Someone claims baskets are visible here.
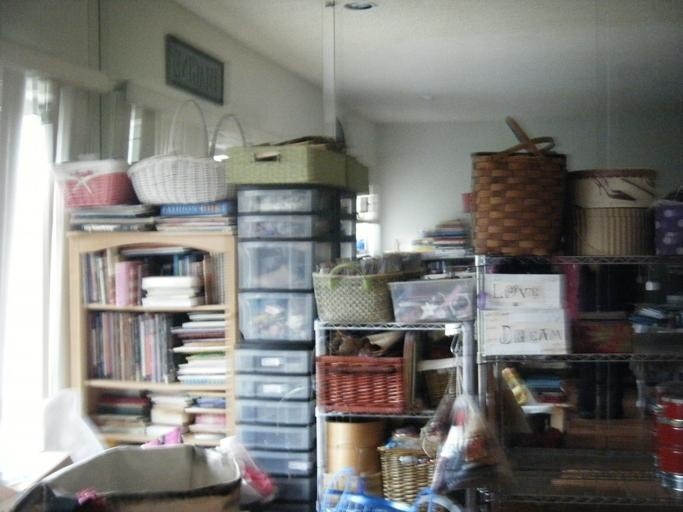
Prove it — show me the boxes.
[313,264,426,324]
[472,116,566,255]
[566,168,657,257]
[61,98,236,206]
[378,444,443,511]
[317,355,405,412]
[427,370,477,408]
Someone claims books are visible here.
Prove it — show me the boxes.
[67,202,238,446]
[413,218,474,255]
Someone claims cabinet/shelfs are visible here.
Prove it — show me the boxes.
[314,319,476,511]
[237,187,357,501]
[474,254,683,511]
[66,228,236,449]
